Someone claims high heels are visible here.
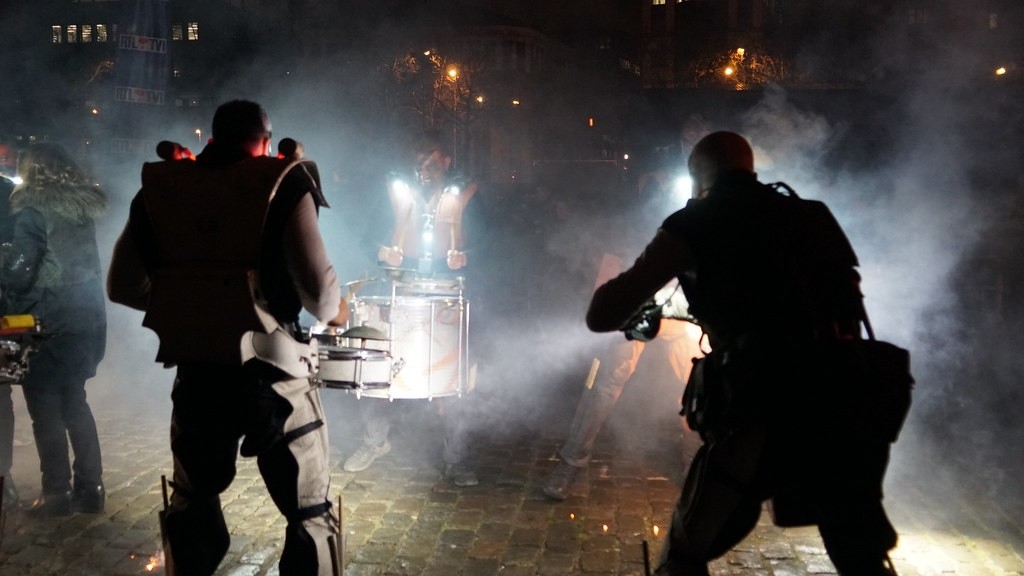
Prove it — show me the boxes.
[71,482,106,514]
[19,481,75,517]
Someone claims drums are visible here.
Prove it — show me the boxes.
[338,273,476,400]
[313,347,394,391]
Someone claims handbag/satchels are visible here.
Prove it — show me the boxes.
[0,329,86,398]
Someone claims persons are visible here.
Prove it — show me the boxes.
[539,105,781,502]
[584,129,901,575]
[106,97,350,576]
[341,131,483,487]
[0,141,108,523]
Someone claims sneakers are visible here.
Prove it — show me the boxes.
[342,436,391,471]
[543,458,580,500]
[445,461,478,487]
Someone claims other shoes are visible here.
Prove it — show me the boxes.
[1,474,19,509]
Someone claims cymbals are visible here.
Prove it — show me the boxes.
[338,325,393,342]
[308,325,351,345]
